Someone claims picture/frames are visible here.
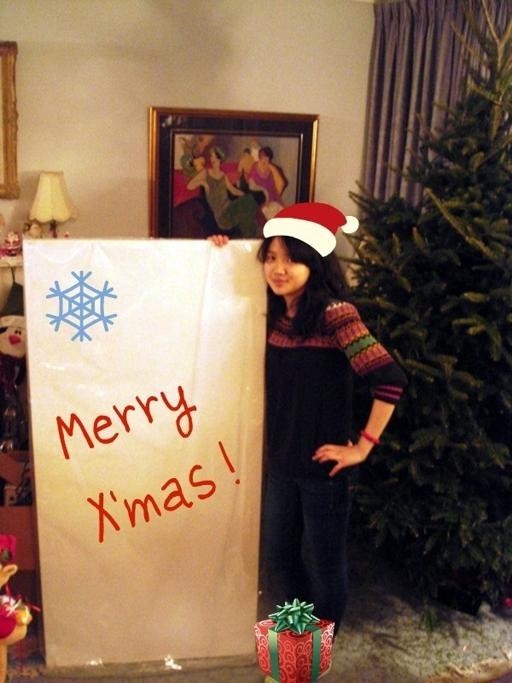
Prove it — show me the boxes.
[0,40,21,200]
[148,105,320,254]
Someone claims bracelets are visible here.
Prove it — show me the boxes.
[358,431,381,445]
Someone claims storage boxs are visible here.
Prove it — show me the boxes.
[0,451,38,576]
[253,614,335,683]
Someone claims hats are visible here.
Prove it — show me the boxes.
[262,202,360,258]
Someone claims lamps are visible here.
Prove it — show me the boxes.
[27,170,77,239]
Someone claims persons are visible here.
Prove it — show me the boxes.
[208,201,407,646]
[186,147,287,237]
